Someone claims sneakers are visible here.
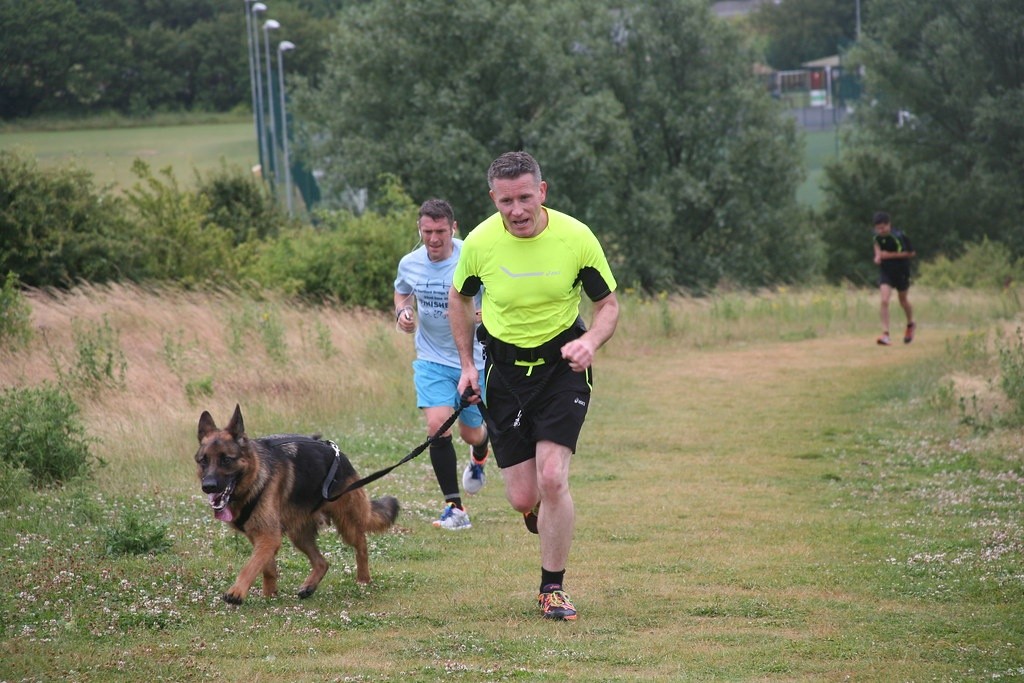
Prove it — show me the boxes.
[540,583,577,621]
[462,443,490,494]
[432,501,472,530]
[522,499,539,535]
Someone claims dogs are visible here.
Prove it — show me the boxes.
[195,404,400,605]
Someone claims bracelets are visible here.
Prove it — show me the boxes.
[397,309,404,321]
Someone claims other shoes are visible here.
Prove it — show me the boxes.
[877,334,890,345]
[904,323,917,343]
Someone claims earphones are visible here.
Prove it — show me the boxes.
[419,229,421,240]
[451,229,455,238]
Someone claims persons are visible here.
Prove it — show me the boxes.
[874,213,917,345]
[448,152,620,620]
[394,200,488,529]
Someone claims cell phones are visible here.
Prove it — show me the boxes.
[404,309,410,321]
